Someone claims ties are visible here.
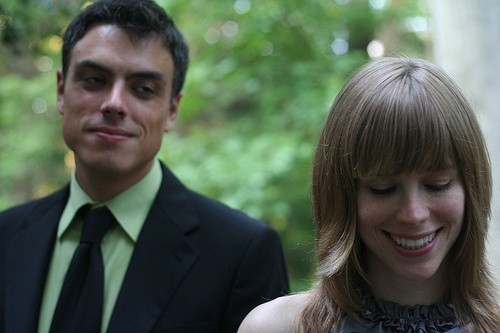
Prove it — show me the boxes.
[49,205,116,333]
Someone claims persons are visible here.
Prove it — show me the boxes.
[238,57,500,333]
[0,0,292,333]
